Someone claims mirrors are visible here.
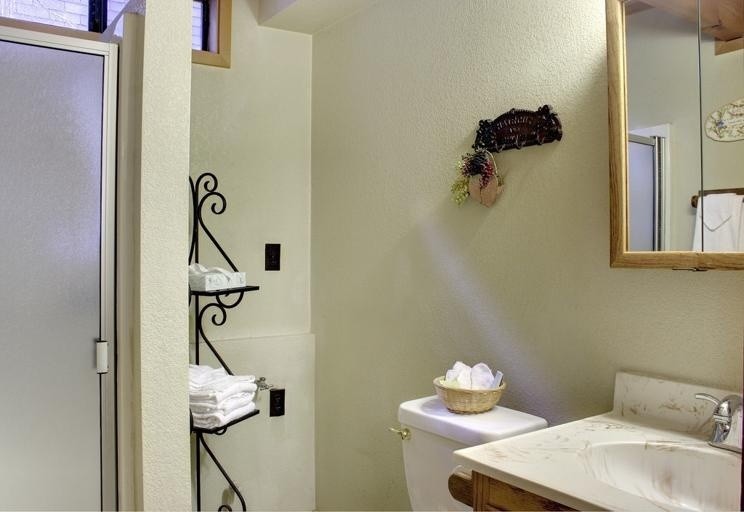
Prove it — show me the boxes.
[604,0,744,272]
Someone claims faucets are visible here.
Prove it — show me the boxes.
[710,395,743,454]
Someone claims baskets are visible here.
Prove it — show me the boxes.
[468,149,503,208]
[433,376,505,414]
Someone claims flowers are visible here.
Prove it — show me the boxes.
[450,146,495,209]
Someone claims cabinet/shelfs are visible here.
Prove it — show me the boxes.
[188,173,261,511]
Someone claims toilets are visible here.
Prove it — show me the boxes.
[389,396,549,512]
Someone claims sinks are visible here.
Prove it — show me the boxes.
[576,442,743,512]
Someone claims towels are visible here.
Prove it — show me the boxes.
[692,193,744,254]
[190,363,258,430]
